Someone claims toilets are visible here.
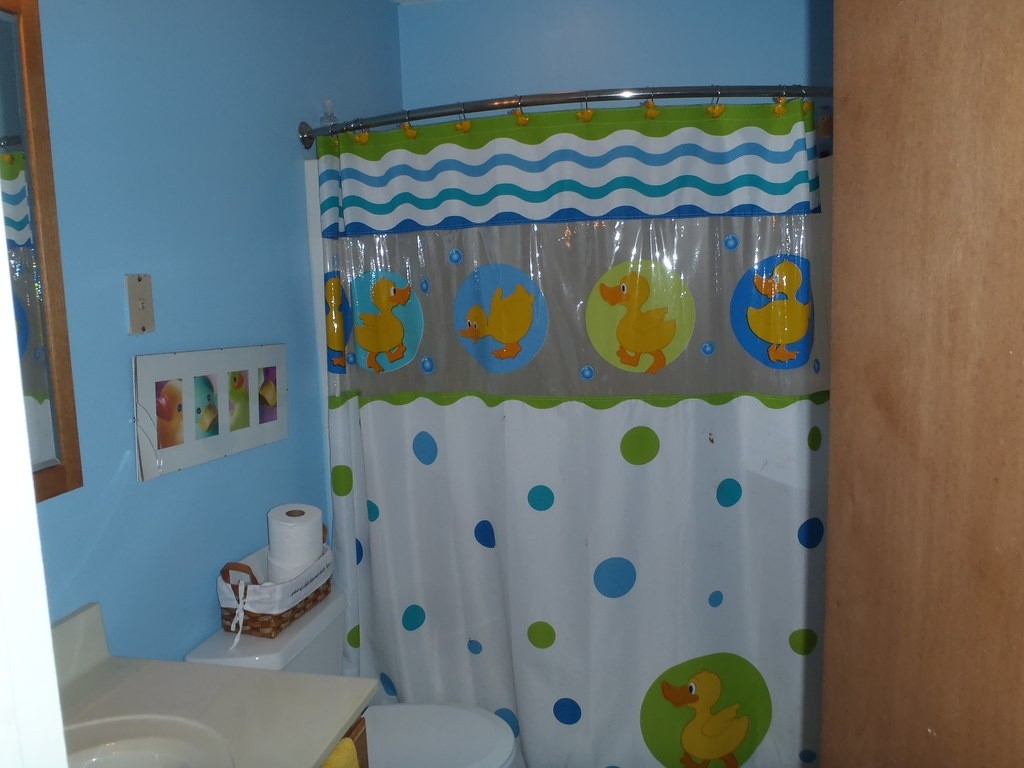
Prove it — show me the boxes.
[184,585,517,768]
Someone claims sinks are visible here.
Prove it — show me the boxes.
[62,711,238,768]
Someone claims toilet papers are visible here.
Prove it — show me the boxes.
[266,503,324,586]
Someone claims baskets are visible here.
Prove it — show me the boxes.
[217,523,333,637]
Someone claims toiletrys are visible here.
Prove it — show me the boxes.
[319,98,339,130]
